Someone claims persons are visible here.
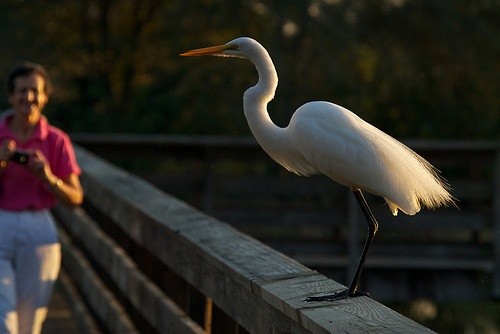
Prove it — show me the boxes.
[0,62,84,334]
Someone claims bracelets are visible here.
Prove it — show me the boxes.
[46,180,63,193]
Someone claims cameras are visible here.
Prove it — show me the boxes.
[9,149,29,164]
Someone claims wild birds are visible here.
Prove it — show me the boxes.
[179,37,462,303]
